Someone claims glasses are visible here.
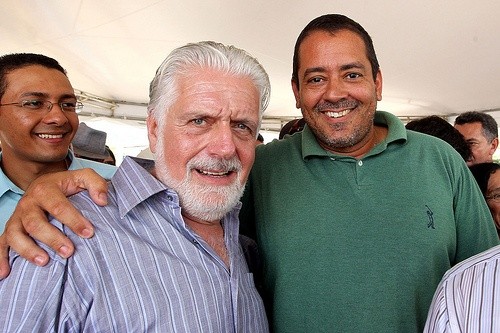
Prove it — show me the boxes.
[0,97,84,115]
[485,192,500,202]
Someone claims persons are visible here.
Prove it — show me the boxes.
[1,40,270,333]
[2,53,117,237]
[72,122,155,170]
[422,243,500,333]
[1,14,500,332]
[254,109,500,238]
